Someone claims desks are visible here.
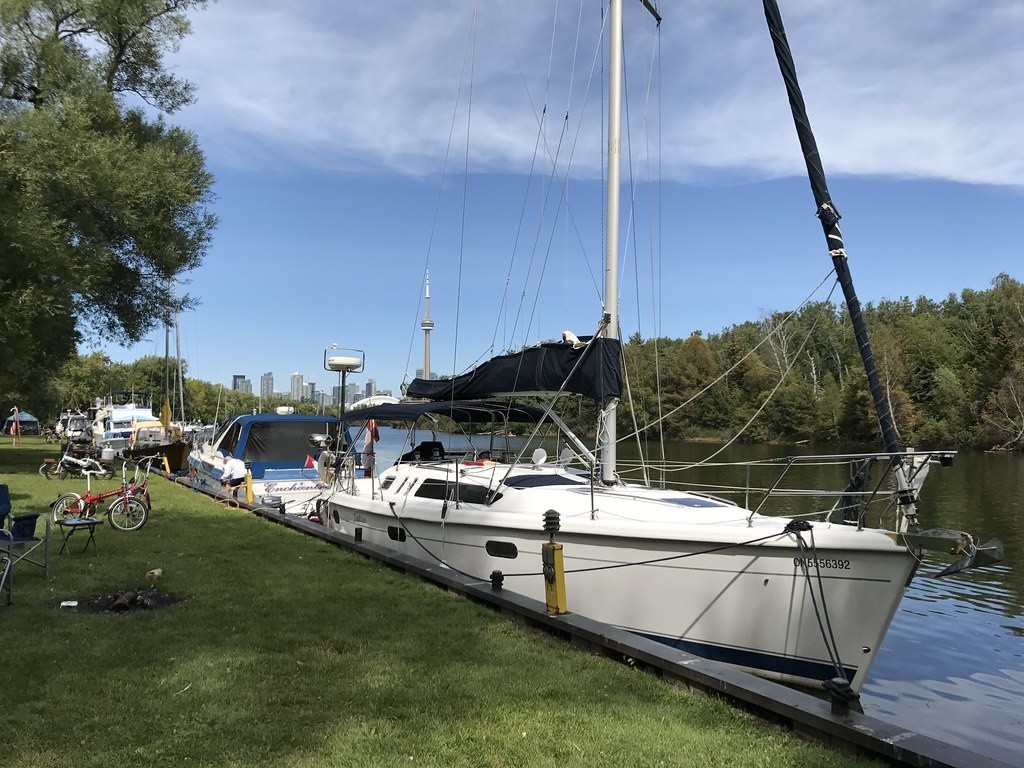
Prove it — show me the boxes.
[70,449,99,480]
[55,518,104,559]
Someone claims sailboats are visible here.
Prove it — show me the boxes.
[120,276,196,473]
[307,0,1006,705]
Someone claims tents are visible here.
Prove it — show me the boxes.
[3,411,40,436]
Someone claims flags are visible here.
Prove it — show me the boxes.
[367,419,380,442]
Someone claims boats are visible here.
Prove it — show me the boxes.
[39,379,222,481]
[186,383,381,521]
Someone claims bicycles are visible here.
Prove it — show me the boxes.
[116,452,165,517]
[39,439,115,482]
[50,451,149,531]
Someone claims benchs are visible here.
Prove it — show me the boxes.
[44,457,56,463]
[99,461,114,465]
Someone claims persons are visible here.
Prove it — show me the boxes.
[219,456,247,501]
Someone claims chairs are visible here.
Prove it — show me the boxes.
[0,484,51,607]
